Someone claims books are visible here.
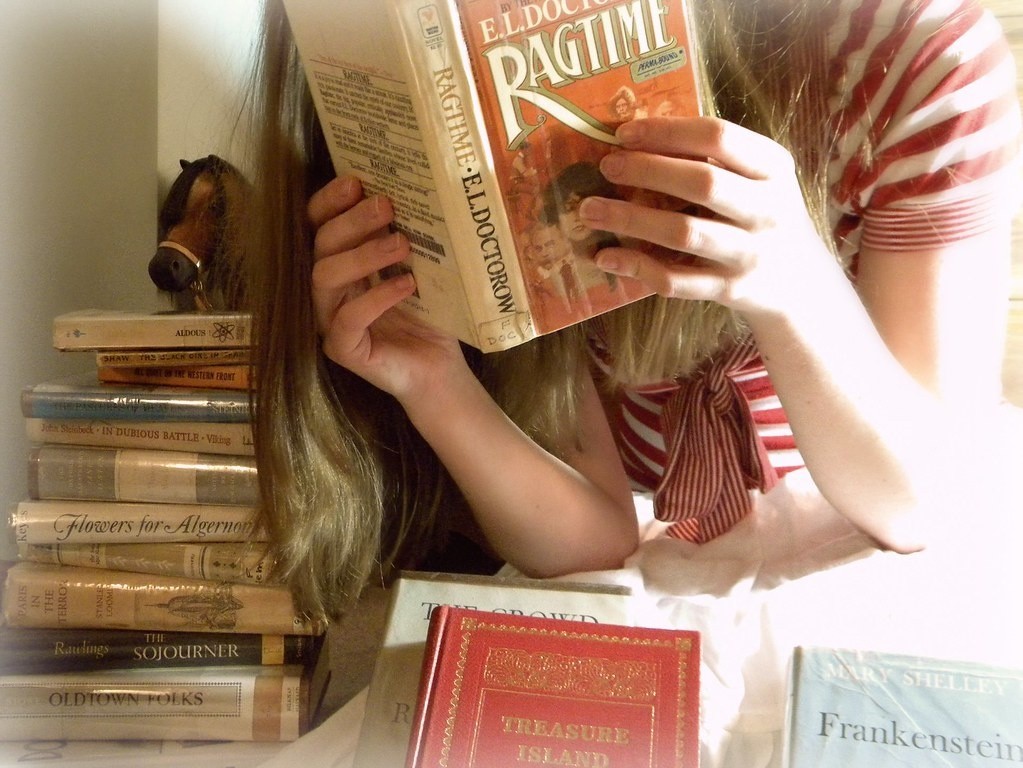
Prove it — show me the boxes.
[791,645,1023,768]
[286,2,736,355]
[0,310,717,768]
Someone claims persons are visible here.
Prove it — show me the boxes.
[244,2,1023,582]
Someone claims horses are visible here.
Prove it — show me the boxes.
[146,154,253,313]
